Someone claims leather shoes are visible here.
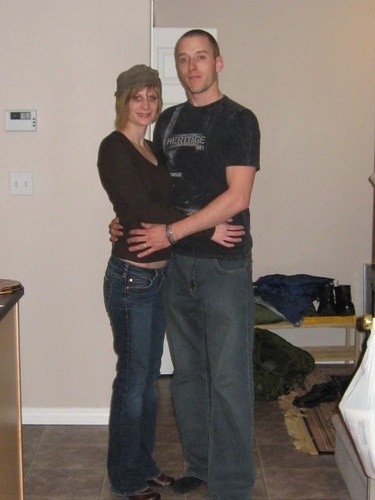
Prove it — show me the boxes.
[148,473,175,487]
[129,487,160,500]
[293,381,337,407]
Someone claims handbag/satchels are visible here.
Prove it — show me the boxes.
[252,326,315,402]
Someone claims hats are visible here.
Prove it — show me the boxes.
[116,65,160,94]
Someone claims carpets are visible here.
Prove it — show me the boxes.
[278,364,359,455]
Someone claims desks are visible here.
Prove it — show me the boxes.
[0,287,24,500]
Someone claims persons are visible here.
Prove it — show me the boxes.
[97,64,246,500]
[107,30,261,500]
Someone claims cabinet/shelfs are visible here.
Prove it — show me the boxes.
[254,313,360,368]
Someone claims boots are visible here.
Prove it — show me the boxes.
[334,284,355,315]
[318,282,335,316]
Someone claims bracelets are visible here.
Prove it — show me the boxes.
[166,224,175,244]
